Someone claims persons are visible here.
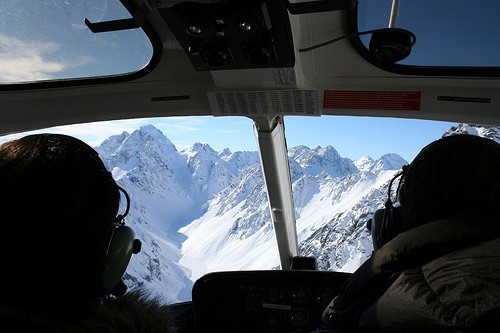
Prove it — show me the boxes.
[0,134,175,333]
[320,134,500,333]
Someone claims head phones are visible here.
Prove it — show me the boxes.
[99,185,141,297]
[366,163,411,250]
